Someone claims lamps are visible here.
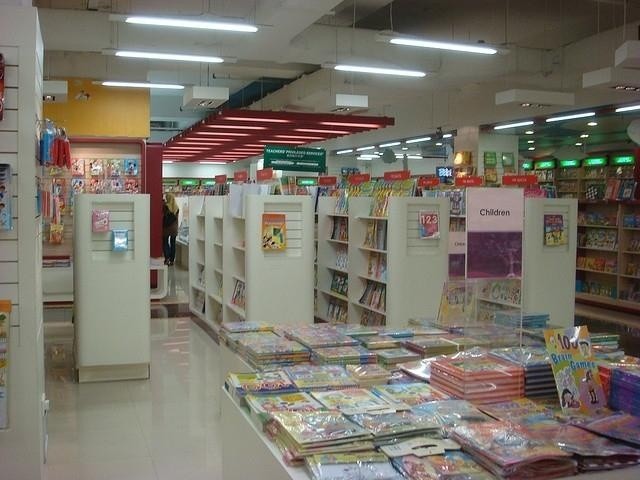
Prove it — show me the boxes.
[375,1,512,57]
[106,0,259,34]
[492,99,640,130]
[454,151,471,164]
[97,17,239,66]
[92,52,214,91]
[318,1,433,80]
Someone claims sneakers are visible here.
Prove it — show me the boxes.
[164,257,170,265]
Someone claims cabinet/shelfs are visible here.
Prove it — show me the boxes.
[162,167,313,333]
[449,167,640,340]
[314,168,448,326]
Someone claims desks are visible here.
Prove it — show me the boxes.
[219,380,640,480]
[213,325,621,372]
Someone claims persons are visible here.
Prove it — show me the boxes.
[161,193,180,266]
